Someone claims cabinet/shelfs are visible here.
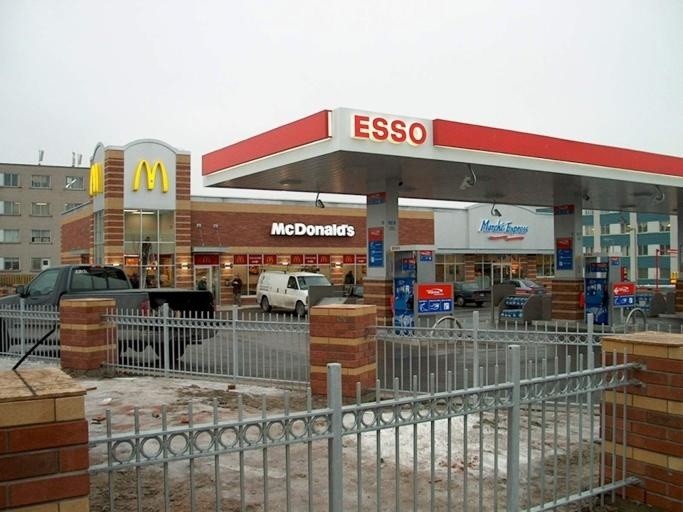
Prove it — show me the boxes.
[623,290,667,316]
[494,293,541,326]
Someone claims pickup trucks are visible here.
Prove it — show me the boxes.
[0,263,218,362]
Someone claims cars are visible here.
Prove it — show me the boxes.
[448,281,490,307]
[506,276,540,291]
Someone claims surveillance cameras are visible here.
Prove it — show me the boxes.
[459,174,471,192]
[494,208,501,218]
[394,177,407,189]
[317,199,324,209]
[583,194,589,200]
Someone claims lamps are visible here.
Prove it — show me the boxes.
[457,164,476,190]
[650,185,665,203]
[314,191,324,209]
[389,176,403,206]
[491,200,501,217]
[581,192,589,202]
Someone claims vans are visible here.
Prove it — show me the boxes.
[255,268,333,318]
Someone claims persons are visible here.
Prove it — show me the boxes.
[345,270,354,283]
[198,276,208,290]
[146,277,151,288]
[131,272,139,289]
[141,236,152,265]
[232,273,242,307]
